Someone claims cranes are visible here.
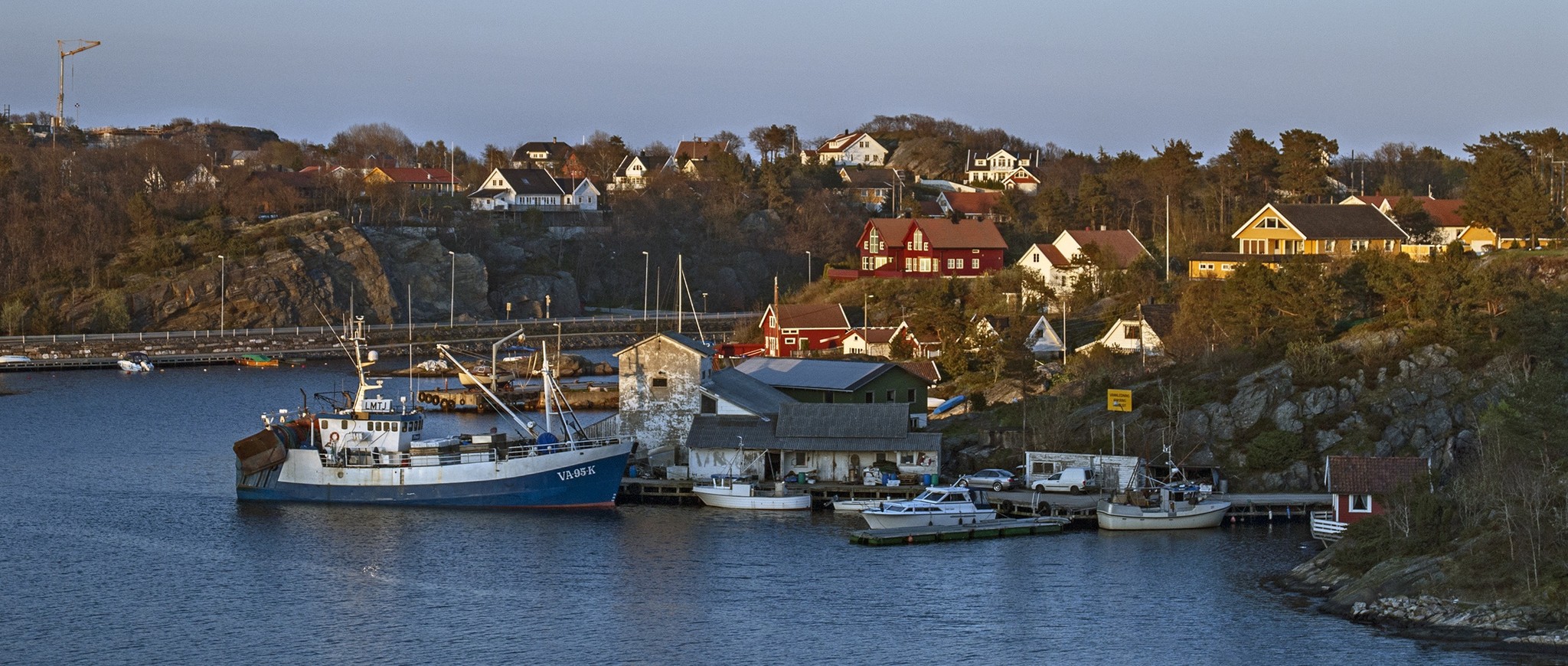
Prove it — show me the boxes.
[56,39,101,127]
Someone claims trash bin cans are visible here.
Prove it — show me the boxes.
[1219,477,1228,493]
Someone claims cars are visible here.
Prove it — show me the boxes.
[1031,466,1096,496]
[957,469,1022,492]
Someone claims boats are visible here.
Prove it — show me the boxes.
[1096,446,1233,531]
[833,484,998,530]
[233,303,639,509]
[691,436,813,510]
[232,353,279,366]
[117,352,154,372]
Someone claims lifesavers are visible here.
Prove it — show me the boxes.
[534,392,538,397]
[330,432,339,441]
[529,393,534,399]
[419,392,425,402]
[479,401,516,411]
[1038,501,1051,516]
[482,394,523,402]
[1002,499,1013,513]
[440,398,448,408]
[629,483,642,495]
[447,400,456,410]
[525,393,529,399]
[425,394,432,404]
[460,399,465,405]
[431,395,439,406]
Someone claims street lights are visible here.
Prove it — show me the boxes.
[218,255,224,337]
[642,251,648,322]
[865,293,874,355]
[805,251,811,286]
[553,322,561,391]
[448,251,455,328]
